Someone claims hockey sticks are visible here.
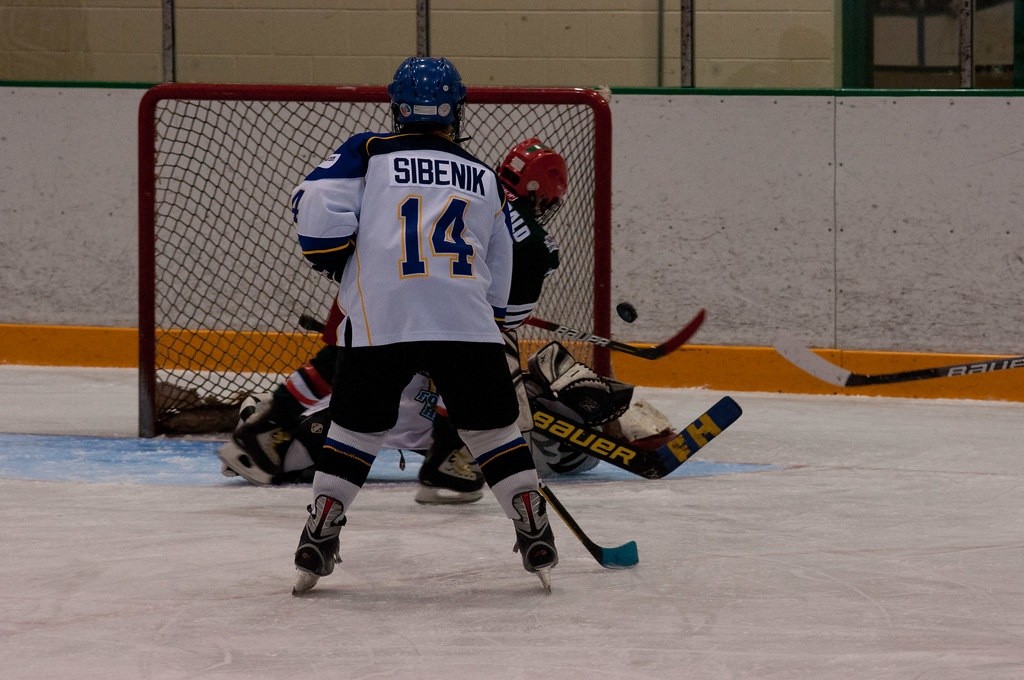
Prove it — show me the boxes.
[523,307,706,360]
[297,313,742,480]
[773,335,1024,387]
[537,475,639,570]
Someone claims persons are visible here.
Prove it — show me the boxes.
[211,139,568,504]
[291,57,560,597]
[236,341,672,479]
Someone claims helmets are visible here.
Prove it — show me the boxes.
[498,138,569,224]
[387,54,467,135]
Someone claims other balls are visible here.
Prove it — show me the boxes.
[616,302,638,323]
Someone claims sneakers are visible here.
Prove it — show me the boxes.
[217,412,291,485]
[292,493,347,597]
[509,490,558,593]
[415,414,485,501]
